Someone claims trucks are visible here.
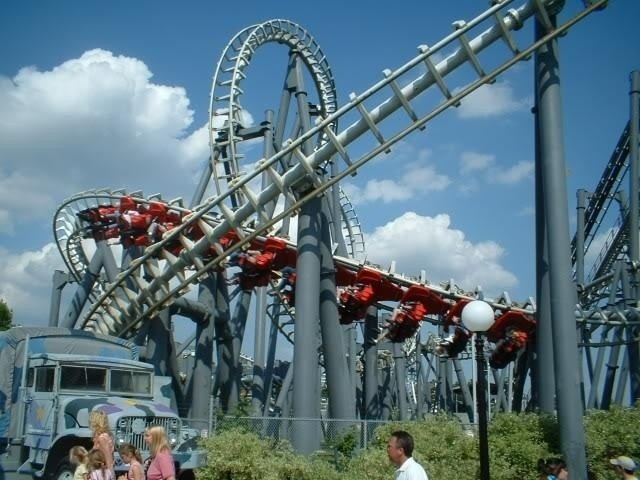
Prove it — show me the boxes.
[0,326,207,480]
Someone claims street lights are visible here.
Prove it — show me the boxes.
[459,300,495,480]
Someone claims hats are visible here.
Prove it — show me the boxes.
[609,456,636,471]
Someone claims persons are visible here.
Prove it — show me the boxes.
[74,209,527,369]
[609,456,640,480]
[538,458,569,480]
[69,408,176,480]
[387,431,429,480]
